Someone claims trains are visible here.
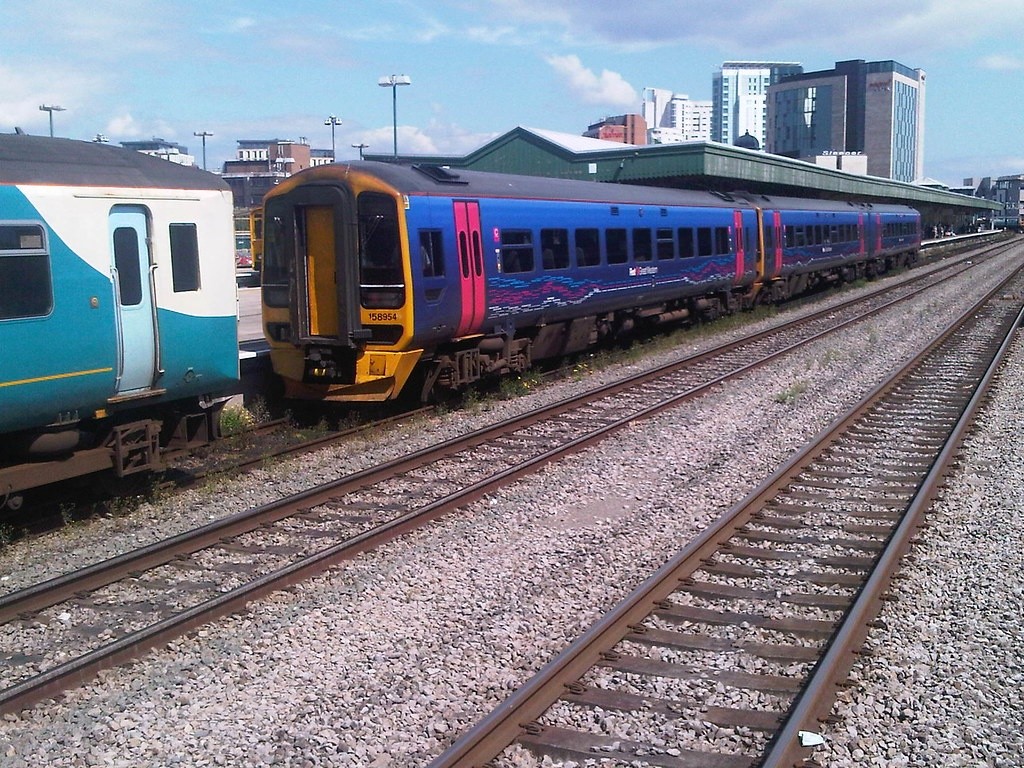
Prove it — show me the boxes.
[984,215,1024,233]
[1,126,244,528]
[264,160,921,407]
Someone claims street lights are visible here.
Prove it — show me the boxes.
[193,131,213,170]
[39,105,68,137]
[351,144,370,161]
[324,111,343,164]
[377,73,411,161]
[274,157,295,183]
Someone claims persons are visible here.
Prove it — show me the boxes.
[930,219,982,239]
